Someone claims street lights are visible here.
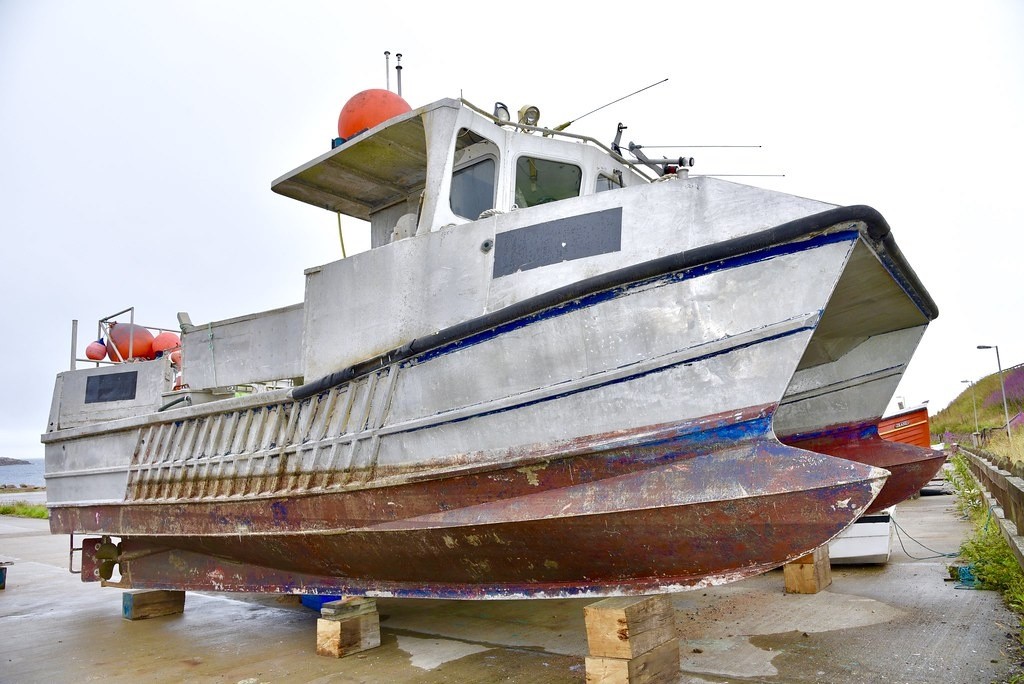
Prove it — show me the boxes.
[961,380,978,433]
[977,345,1012,443]
[896,396,905,406]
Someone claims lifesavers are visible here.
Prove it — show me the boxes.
[161,352,182,391]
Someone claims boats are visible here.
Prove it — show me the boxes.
[878,404,931,449]
[40,50,950,599]
[828,503,899,565]
